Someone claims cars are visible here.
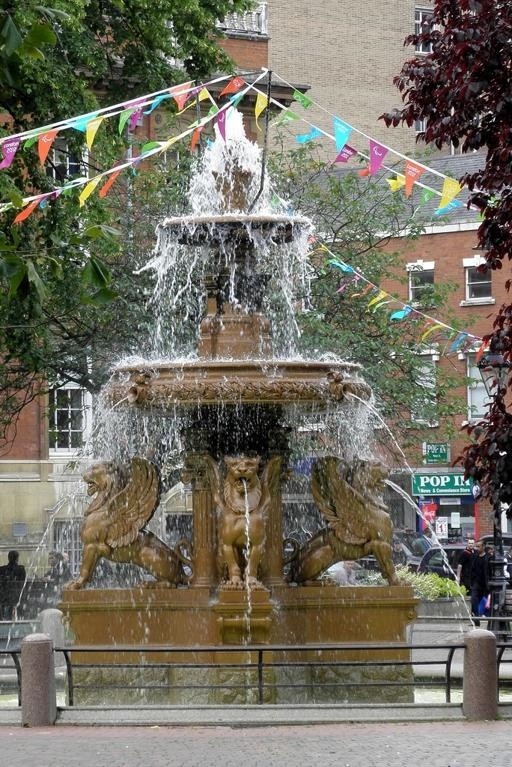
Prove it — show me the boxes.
[475,534,512,564]
[416,546,512,593]
[355,529,434,573]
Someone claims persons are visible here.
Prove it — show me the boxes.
[0,550,28,620]
[87,524,475,588]
[40,548,73,609]
[456,536,512,625]
